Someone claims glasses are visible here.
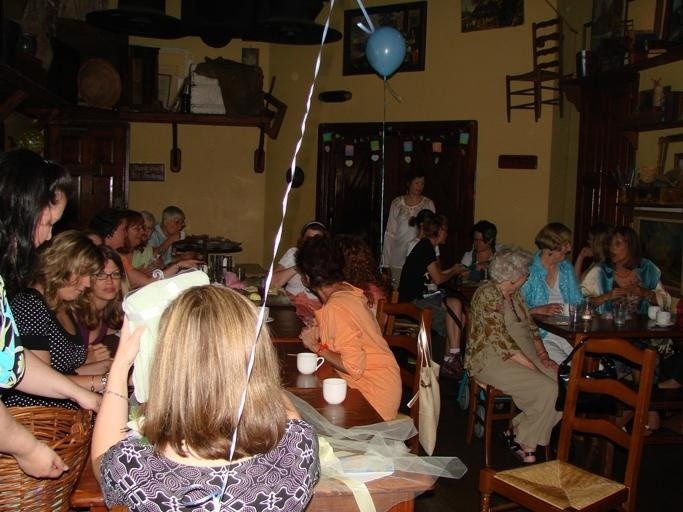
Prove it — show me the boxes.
[93,270,122,280]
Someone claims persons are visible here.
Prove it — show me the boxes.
[1,149,102,478]
[270,222,330,300]
[520,222,603,381]
[454,219,498,282]
[308,235,384,325]
[131,211,156,278]
[463,241,564,467]
[67,244,124,345]
[90,285,321,511]
[116,210,155,288]
[2,230,133,426]
[405,209,440,292]
[575,225,601,297]
[88,208,203,288]
[149,206,186,268]
[295,234,402,422]
[397,214,465,376]
[381,172,439,289]
[581,224,671,436]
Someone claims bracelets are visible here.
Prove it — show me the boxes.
[103,390,130,403]
[536,349,548,354]
[91,375,95,392]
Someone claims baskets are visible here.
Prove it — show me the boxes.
[1,405,93,511]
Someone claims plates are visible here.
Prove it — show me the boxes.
[260,317,274,323]
[656,322,674,327]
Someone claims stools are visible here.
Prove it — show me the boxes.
[620,388,683,443]
[465,377,549,467]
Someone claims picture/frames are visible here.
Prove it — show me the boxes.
[342,2,426,76]
[659,135,682,175]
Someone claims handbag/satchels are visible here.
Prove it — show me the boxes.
[555,333,618,415]
[418,321,440,456]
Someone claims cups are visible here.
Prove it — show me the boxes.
[295,351,324,375]
[613,301,627,327]
[232,266,246,281]
[569,303,582,327]
[656,311,672,324]
[648,305,661,320]
[257,306,269,320]
[321,377,348,405]
[581,298,594,321]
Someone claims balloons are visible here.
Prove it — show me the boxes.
[365,27,406,77]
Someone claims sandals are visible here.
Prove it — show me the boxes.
[513,450,537,469]
[504,427,516,449]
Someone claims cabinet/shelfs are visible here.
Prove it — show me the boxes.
[562,52,682,298]
[1,108,127,227]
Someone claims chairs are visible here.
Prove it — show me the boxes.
[376,298,430,456]
[505,19,562,121]
[478,334,655,511]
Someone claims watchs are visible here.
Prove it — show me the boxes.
[101,372,109,393]
[317,343,328,355]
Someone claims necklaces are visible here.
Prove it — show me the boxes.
[502,292,521,322]
